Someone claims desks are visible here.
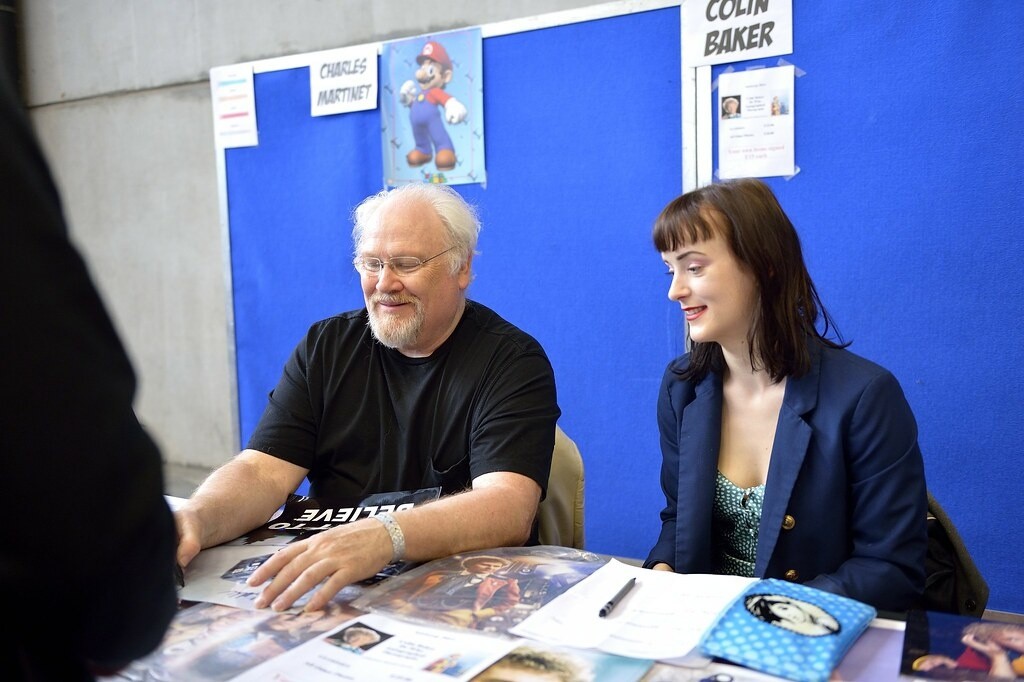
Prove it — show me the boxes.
[98,496,1024,682]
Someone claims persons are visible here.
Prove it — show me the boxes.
[208,597,338,665]
[469,650,582,682]
[326,627,379,655]
[379,555,520,629]
[0,0,178,681]
[430,652,462,677]
[641,178,927,614]
[164,185,561,610]
[960,622,1024,682]
[772,96,781,116]
[722,97,741,119]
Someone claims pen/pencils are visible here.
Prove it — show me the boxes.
[599,577,637,617]
[176,563,185,588]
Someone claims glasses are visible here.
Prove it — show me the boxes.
[353,245,455,277]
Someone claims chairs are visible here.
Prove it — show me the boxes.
[924,496,991,617]
[536,424,584,552]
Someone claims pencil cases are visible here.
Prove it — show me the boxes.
[697,577,879,682]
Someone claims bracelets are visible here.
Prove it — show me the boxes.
[369,513,404,564]
